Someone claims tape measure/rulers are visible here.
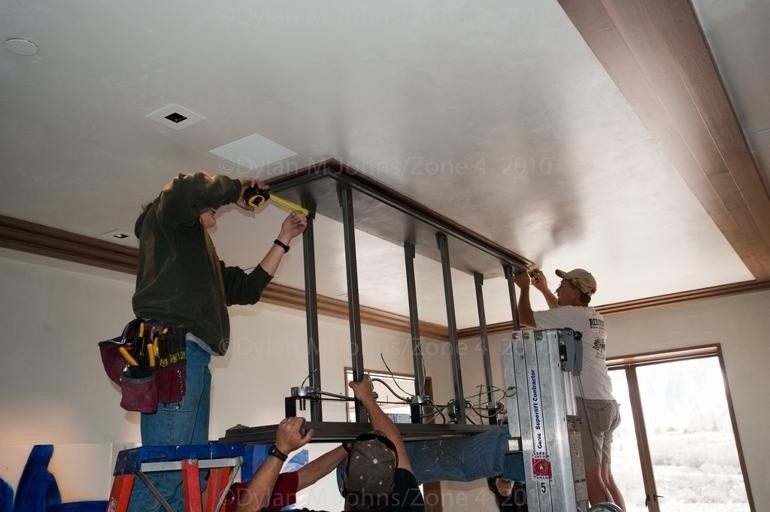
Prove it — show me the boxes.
[243,184,309,217]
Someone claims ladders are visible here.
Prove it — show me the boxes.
[107,443,243,512]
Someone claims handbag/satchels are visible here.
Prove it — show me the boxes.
[98,319,187,413]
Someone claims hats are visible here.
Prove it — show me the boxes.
[555,269,597,296]
[343,430,396,494]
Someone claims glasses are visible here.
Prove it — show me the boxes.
[345,434,399,477]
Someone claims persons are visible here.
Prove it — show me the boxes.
[487,415,528,512]
[514,266,626,512]
[222,441,352,512]
[238,374,426,511]
[126,171,310,512]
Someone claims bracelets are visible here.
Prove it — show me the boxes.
[273,238,289,253]
[342,442,351,454]
[268,446,288,462]
